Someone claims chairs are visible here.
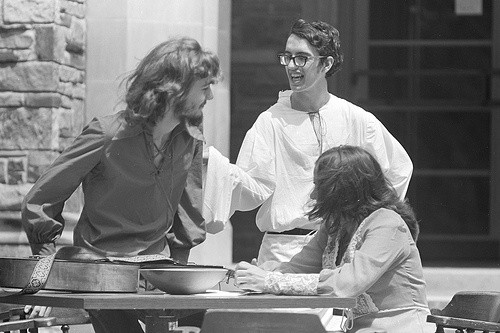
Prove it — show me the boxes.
[333,291,500,333]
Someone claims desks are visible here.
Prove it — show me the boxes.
[0,291,357,333]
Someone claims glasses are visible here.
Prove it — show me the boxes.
[278,53,324,67]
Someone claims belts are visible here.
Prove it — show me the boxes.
[266,228,315,235]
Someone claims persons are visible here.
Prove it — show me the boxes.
[21,37,224,333]
[234,144,432,333]
[203,15,414,264]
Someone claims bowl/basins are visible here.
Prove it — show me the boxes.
[139,267,229,294]
